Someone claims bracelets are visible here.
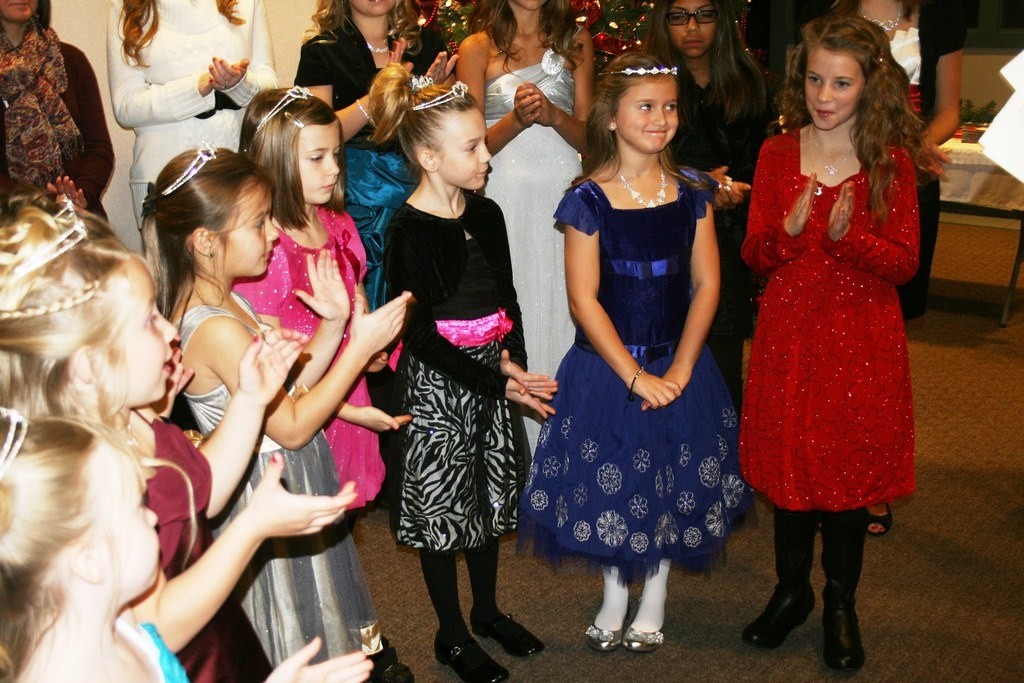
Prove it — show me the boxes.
[627,366,645,402]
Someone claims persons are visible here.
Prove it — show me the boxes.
[106,0,280,295]
[0,194,361,659]
[143,147,412,682]
[364,61,559,682]
[0,0,117,232]
[292,0,456,553]
[635,0,770,399]
[737,15,947,675]
[228,85,418,682]
[517,50,752,655]
[810,0,967,536]
[3,410,375,683]
[456,0,598,462]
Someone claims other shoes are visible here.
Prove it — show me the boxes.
[865,503,893,537]
[622,614,664,652]
[585,602,629,650]
[362,635,413,683]
[470,609,545,658]
[434,629,510,683]
[817,511,822,530]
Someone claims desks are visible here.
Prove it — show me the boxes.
[935,138,1024,328]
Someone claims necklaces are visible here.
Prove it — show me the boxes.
[610,154,667,208]
[367,41,389,54]
[858,8,903,30]
[808,121,856,175]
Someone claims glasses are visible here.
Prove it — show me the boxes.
[666,9,718,26]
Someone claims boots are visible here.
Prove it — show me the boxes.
[742,509,819,649]
[821,507,869,674]
